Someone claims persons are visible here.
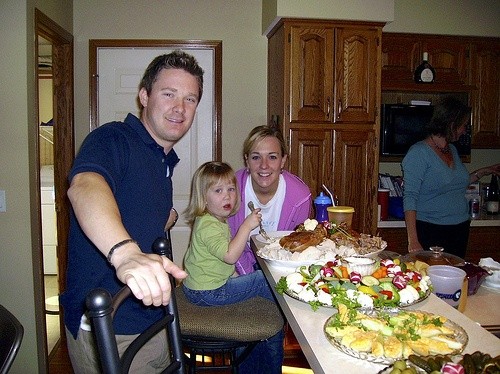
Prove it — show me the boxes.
[60,49,204,374]
[227,125,313,374]
[401,98,500,259]
[182,161,277,306]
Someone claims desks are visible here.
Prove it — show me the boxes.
[250,234,500,374]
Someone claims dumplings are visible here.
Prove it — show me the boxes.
[326,303,465,360]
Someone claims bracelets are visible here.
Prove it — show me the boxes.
[474,171,480,180]
[107,239,137,263]
[172,208,178,223]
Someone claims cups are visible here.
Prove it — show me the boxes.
[427,264,466,309]
[327,206,355,232]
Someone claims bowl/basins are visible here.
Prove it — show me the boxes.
[407,247,490,295]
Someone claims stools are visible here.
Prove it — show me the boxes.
[86,220,284,374]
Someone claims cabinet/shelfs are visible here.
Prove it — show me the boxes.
[265,19,500,261]
[41,165,60,274]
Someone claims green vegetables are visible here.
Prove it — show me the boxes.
[275,264,444,340]
[326,220,340,234]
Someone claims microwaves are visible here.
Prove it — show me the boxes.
[381,103,471,164]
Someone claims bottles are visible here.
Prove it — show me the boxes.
[414,51,436,85]
[486,172,500,215]
[314,192,331,222]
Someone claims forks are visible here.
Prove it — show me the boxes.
[248,201,270,239]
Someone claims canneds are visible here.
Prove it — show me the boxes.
[469,198,479,217]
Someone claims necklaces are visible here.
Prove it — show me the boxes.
[431,135,449,154]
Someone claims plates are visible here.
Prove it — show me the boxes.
[255,230,295,243]
[257,239,387,267]
[324,306,470,365]
[274,266,431,306]
[376,354,467,374]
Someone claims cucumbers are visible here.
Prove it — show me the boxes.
[407,353,450,374]
